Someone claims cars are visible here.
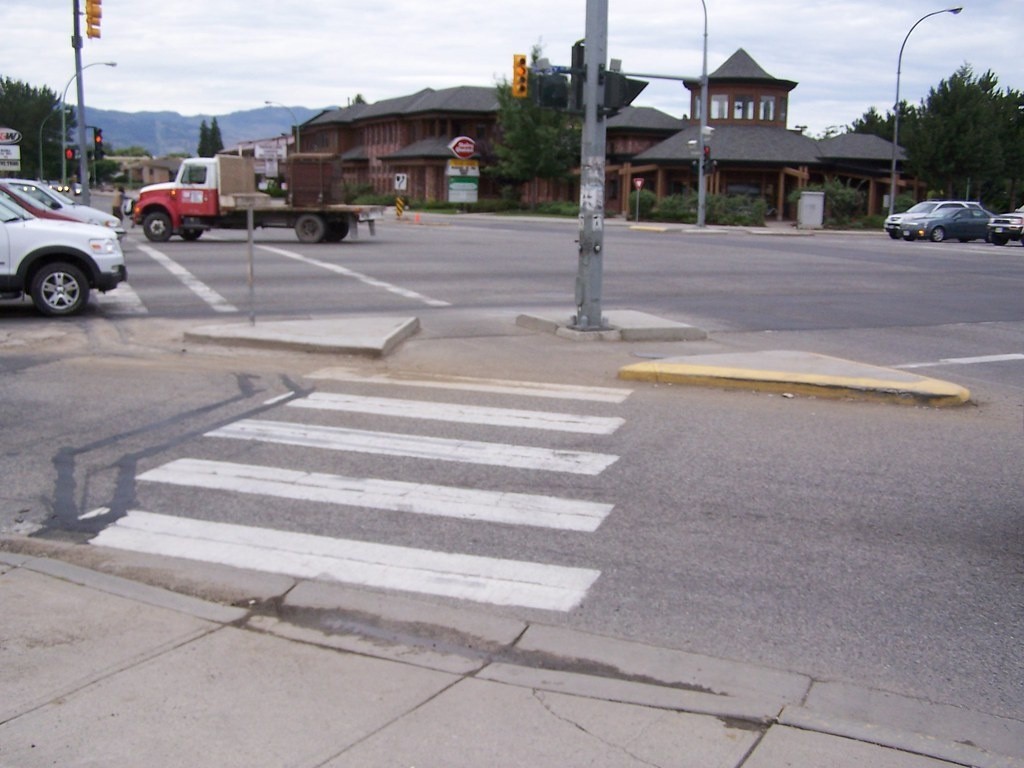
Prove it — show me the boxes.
[0,179,128,317]
[884,200,997,242]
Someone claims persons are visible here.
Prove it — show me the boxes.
[112,187,132,223]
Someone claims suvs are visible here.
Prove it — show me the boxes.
[987,205,1024,246]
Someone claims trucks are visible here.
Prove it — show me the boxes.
[130,154,386,244]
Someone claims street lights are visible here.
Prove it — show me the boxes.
[889,7,962,216]
[265,101,299,153]
[62,61,118,188]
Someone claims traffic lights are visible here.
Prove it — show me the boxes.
[86,0,102,38]
[512,54,528,97]
[96,129,105,160]
[703,146,712,175]
[65,148,75,161]
